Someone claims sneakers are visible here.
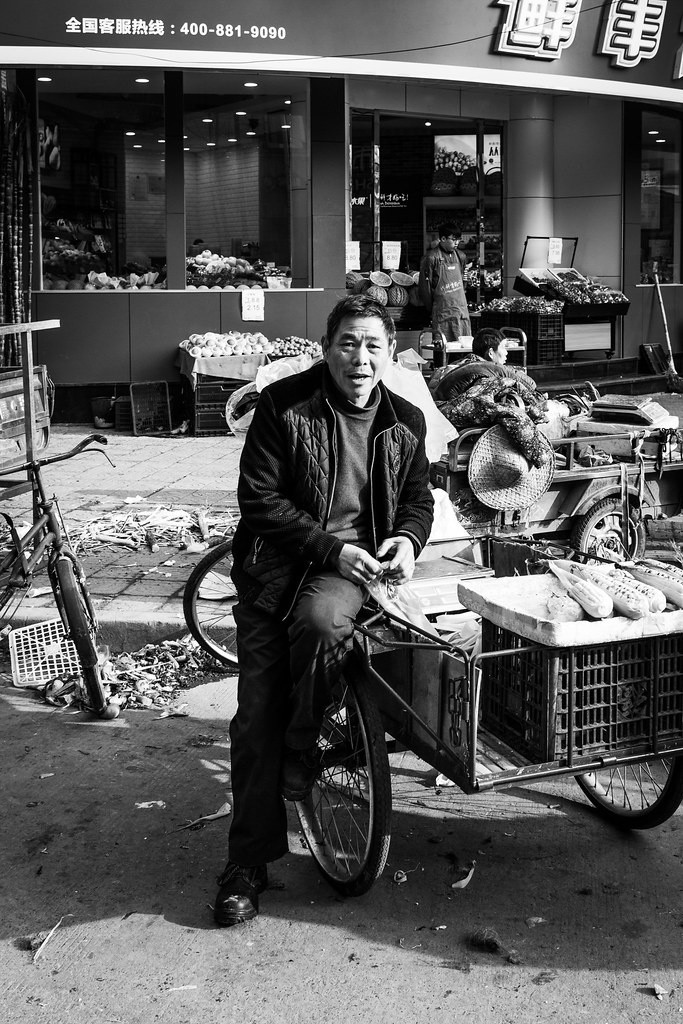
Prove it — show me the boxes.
[273,744,318,801]
[214,860,268,926]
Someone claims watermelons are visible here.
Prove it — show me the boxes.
[346,272,420,306]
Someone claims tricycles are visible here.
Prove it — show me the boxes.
[430,390,683,575]
[182,527,683,896]
[0,431,109,721]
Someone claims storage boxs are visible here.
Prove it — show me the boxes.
[576,414,680,459]
[458,572,683,764]
[468,275,632,365]
[114,344,312,437]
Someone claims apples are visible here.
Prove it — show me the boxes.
[182,332,270,358]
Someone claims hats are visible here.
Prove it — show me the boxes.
[466,422,556,512]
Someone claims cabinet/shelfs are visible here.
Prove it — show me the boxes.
[417,326,527,380]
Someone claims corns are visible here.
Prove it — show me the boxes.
[546,560,683,621]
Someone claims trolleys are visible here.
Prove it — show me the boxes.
[417,327,528,383]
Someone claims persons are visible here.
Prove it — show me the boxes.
[418,223,472,369]
[428,329,508,400]
[213,295,436,922]
